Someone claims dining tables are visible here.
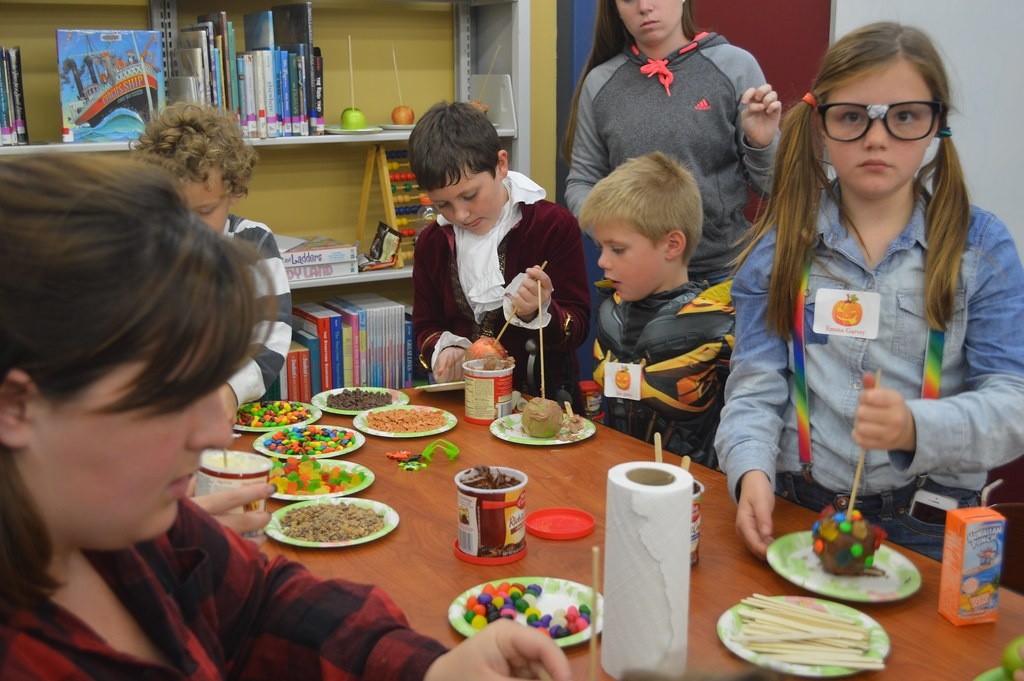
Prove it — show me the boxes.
[193,379,1024,681]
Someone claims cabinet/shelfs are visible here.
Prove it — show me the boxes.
[0,0,533,295]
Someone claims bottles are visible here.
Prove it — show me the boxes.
[414,196,437,243]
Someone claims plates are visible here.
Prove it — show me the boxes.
[231,400,323,432]
[253,425,365,459]
[381,125,416,129]
[449,577,608,648]
[353,405,459,437]
[767,529,918,603]
[311,387,410,415]
[263,460,375,500]
[716,595,889,679]
[325,126,380,134]
[490,413,597,446]
[489,123,498,127]
[263,497,400,548]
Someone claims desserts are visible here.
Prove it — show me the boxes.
[811,504,887,575]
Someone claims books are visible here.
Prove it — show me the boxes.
[264,291,428,404]
[0,45,29,147]
[169,0,325,139]
[56,29,166,144]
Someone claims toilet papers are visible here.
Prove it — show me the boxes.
[601,460,694,681]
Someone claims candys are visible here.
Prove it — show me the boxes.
[464,581,590,640]
[235,400,368,495]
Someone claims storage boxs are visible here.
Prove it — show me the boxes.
[281,234,357,267]
[286,261,359,282]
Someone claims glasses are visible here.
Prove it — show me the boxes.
[816,100,946,142]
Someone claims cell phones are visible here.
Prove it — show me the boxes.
[909,489,958,526]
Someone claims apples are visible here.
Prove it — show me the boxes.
[464,337,508,360]
[1001,635,1024,677]
[521,397,564,439]
[390,106,414,125]
[340,108,366,130]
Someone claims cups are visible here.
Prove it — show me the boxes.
[686,480,704,568]
[194,450,271,543]
[462,358,514,426]
[455,466,527,558]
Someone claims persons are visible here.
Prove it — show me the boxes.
[564,0,782,286]
[713,23,1024,564]
[579,152,736,469]
[127,101,293,404]
[406,102,592,400]
[186,474,274,548]
[0,152,575,681]
